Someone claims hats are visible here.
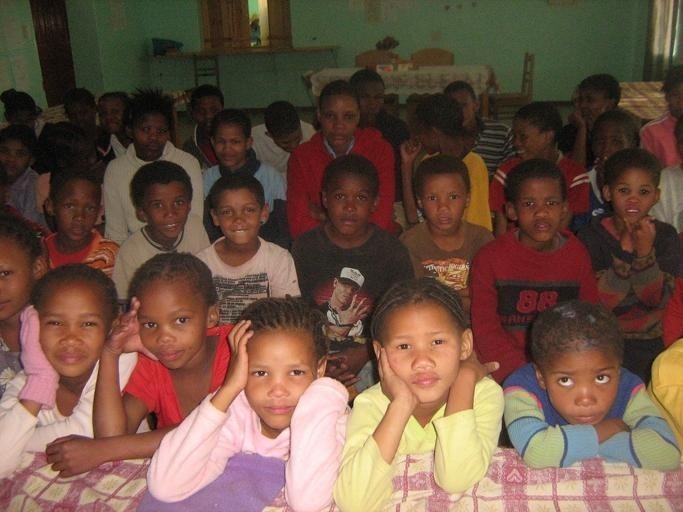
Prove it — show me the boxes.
[337,267,365,291]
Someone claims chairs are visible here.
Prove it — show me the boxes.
[192,53,223,91]
[353,47,407,69]
[488,51,536,118]
[410,46,456,65]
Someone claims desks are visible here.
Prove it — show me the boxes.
[311,64,488,119]
[613,77,667,136]
[0,443,681,512]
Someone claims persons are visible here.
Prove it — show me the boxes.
[644,336,682,454]
[333,277,504,512]
[0,263,153,481]
[1,64,683,407]
[46,251,242,480]
[500,298,682,473]
[145,295,350,512]
[248,13,262,45]
